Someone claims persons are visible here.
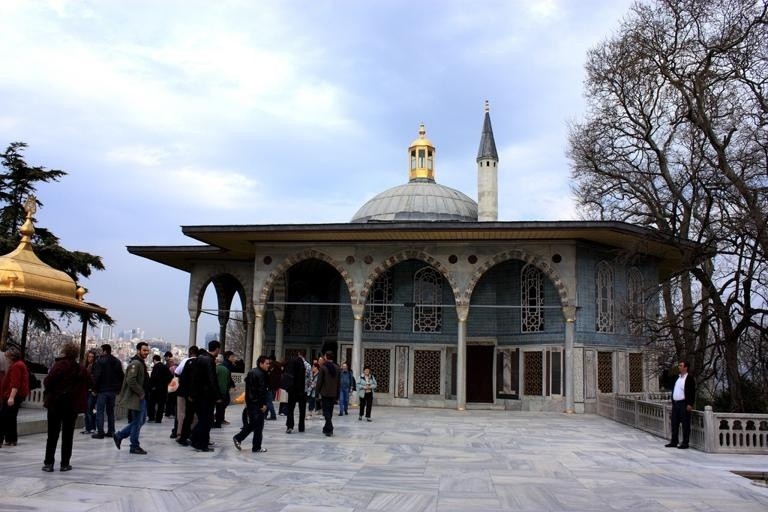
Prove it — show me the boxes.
[662,359,696,448]
[356,367,377,422]
[1,340,29,447]
[233,347,356,452]
[42,341,91,471]
[80,340,245,455]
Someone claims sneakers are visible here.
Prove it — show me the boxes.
[43,464,54,472]
[81,429,147,455]
[230,437,242,450]
[255,448,267,452]
[169,430,215,451]
[60,464,72,471]
